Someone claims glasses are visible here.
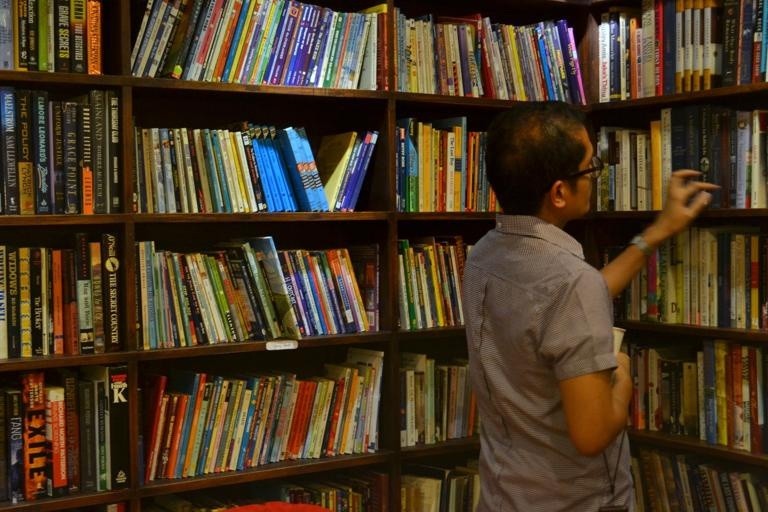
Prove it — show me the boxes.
[571,156,604,178]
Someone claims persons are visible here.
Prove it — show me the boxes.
[460,110,722,512]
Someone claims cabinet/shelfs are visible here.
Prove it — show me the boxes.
[0,0,768,511]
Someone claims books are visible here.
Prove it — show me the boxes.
[393,99,768,213]
[0,228,384,348]
[395,217,762,331]
[395,341,768,511]
[0,357,383,511]
[0,0,390,90]
[391,0,768,105]
[1,85,390,214]
